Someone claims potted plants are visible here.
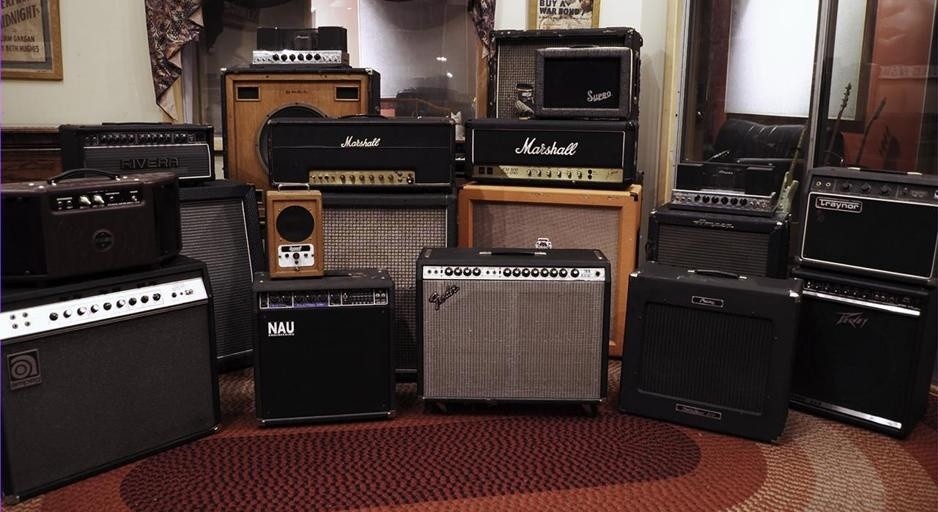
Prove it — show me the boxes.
[526,0,604,32]
[2,1,66,84]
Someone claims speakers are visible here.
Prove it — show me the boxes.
[265,190,326,280]
[59,121,217,185]
[619,158,799,443]
[322,191,457,384]
[785,160,937,438]
[1,127,64,181]
[178,176,266,370]
[253,268,398,429]
[219,66,382,188]
[0,165,183,290]
[2,252,222,508]
[266,114,457,191]
[415,241,612,407]
[459,45,642,361]
